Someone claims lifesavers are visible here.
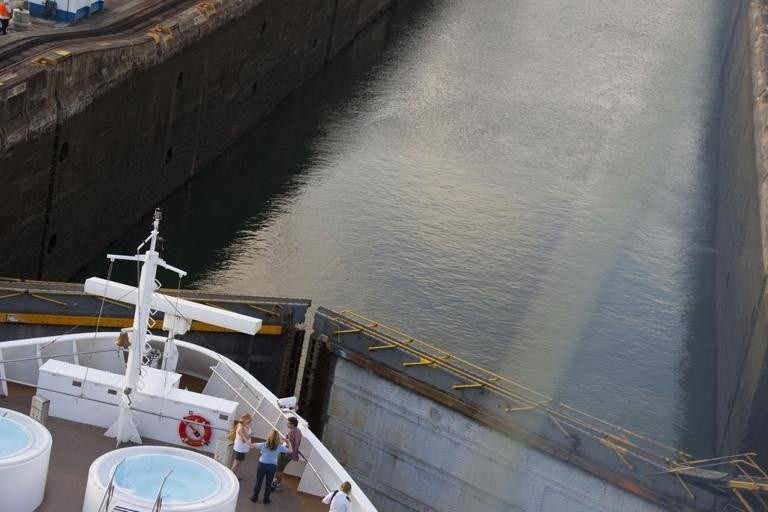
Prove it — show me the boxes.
[180,415,212,447]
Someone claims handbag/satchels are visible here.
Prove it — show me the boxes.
[227,424,237,441]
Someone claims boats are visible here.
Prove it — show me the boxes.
[0,204,380,511]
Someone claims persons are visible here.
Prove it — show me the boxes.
[238,429,294,505]
[271,417,303,491]
[320,481,353,512]
[230,413,255,480]
[0,0,13,35]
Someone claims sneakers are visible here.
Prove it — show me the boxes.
[271,481,277,488]
[274,484,281,491]
